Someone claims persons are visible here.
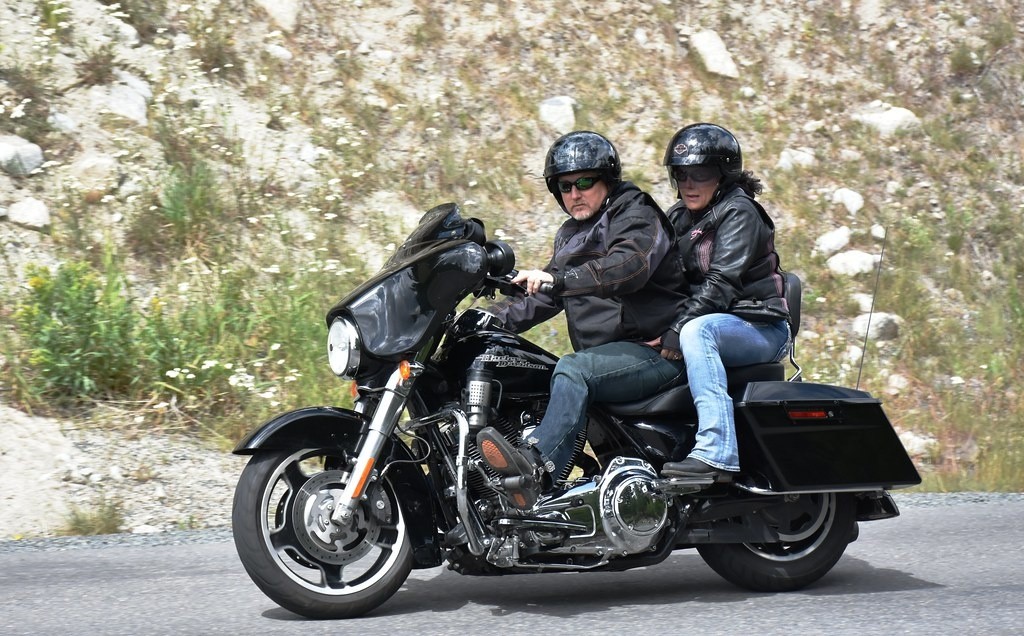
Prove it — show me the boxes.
[477,131,691,510]
[659,123,793,484]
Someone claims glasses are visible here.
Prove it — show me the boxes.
[555,176,602,193]
[672,169,719,182]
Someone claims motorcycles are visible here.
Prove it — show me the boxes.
[230,202,924,622]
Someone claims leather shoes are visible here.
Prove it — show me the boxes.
[478,427,549,509]
[662,458,732,483]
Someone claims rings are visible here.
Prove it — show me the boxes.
[674,354,678,359]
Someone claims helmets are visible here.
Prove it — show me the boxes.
[543,130,622,216]
[663,123,742,178]
